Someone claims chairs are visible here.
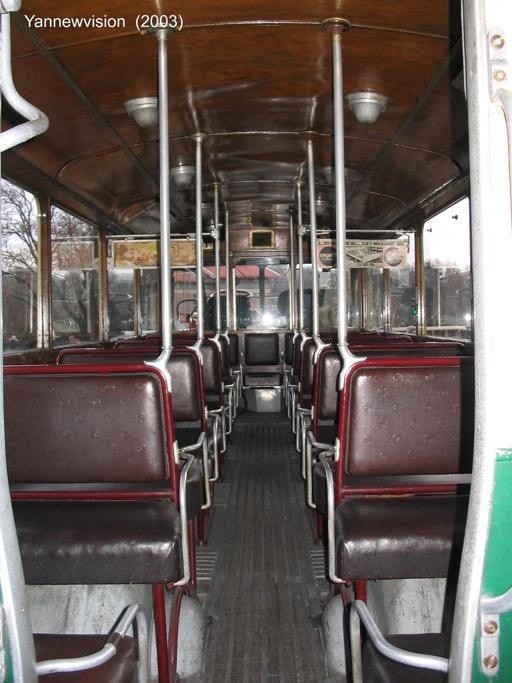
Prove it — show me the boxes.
[244,334,286,415]
[347,601,451,683]
[36,604,151,683]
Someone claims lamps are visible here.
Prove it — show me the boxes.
[345,90,387,123]
[125,97,158,128]
[172,163,195,188]
[313,193,330,215]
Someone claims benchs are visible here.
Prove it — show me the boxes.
[4,361,197,599]
[284,329,474,546]
[54,333,241,546]
[318,355,476,602]
